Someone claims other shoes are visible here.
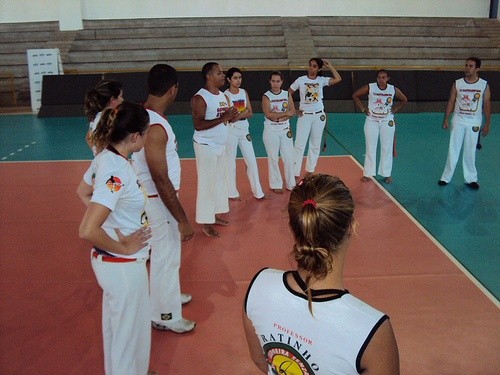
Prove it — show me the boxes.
[465,182,479,190]
[437,180,449,186]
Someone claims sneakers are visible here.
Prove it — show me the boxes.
[180,293,192,305]
[152,316,196,333]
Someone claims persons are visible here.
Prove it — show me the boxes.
[262,69,299,196]
[438,56,493,191]
[76,101,155,375]
[189,61,239,239]
[130,64,195,334]
[241,173,401,375]
[351,68,409,184]
[82,77,124,158]
[288,57,342,185]
[223,66,275,201]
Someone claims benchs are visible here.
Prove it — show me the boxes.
[0,15,500,105]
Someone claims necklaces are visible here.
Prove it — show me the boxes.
[291,272,345,298]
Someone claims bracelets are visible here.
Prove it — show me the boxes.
[361,108,366,113]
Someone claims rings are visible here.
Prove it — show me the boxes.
[230,108,233,110]
